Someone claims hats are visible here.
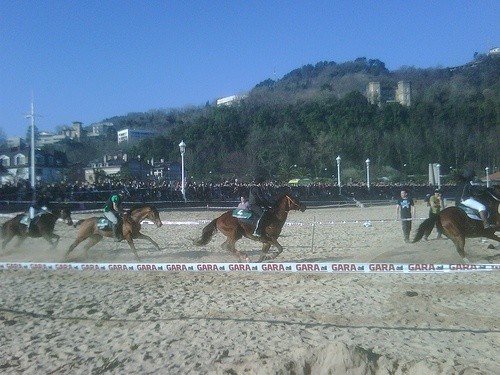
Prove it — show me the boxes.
[434,189,441,193]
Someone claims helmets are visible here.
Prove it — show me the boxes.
[38,194,47,199]
[119,190,128,194]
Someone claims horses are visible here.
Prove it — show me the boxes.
[193,192,307,263]
[65,203,163,263]
[410,194,500,263]
[1,203,73,252]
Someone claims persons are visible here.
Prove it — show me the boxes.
[397,189,415,242]
[249,177,271,237]
[0,177,460,208]
[238,197,250,210]
[424,188,444,241]
[103,188,128,241]
[461,175,490,230]
[24,187,54,231]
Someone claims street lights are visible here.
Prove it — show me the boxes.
[364,157,371,191]
[335,155,343,195]
[436,163,441,190]
[485,166,491,188]
[178,139,187,195]
[24,104,37,188]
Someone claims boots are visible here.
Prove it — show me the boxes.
[26,218,34,233]
[112,224,122,242]
[252,210,268,237]
[479,210,496,229]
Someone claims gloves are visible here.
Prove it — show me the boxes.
[41,207,47,210]
